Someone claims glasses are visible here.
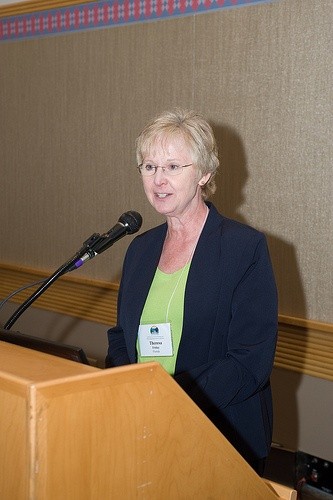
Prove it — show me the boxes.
[137,161,194,177]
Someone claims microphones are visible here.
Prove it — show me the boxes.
[69,210,143,271]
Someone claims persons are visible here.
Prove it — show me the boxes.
[103,106,280,480]
